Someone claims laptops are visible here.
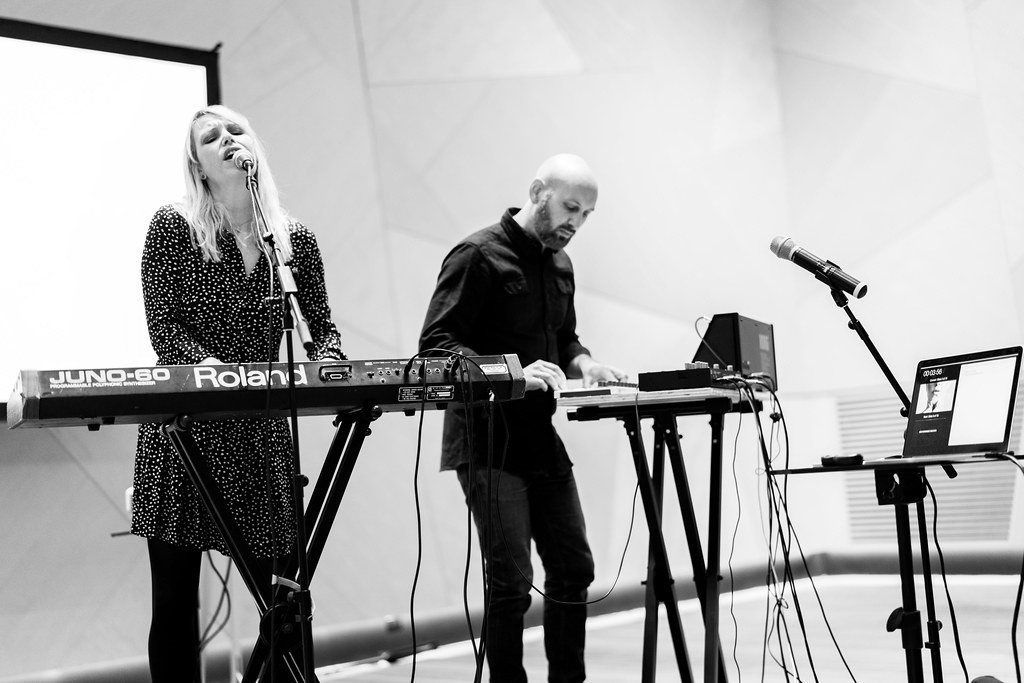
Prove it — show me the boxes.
[862,346,1023,463]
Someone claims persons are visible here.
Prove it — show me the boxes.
[420,155,628,683]
[132,105,345,682]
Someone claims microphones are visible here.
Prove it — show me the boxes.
[232,149,255,169]
[770,236,867,299]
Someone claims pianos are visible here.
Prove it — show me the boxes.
[6,347,526,683]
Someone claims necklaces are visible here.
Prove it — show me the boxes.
[232,219,252,232]
